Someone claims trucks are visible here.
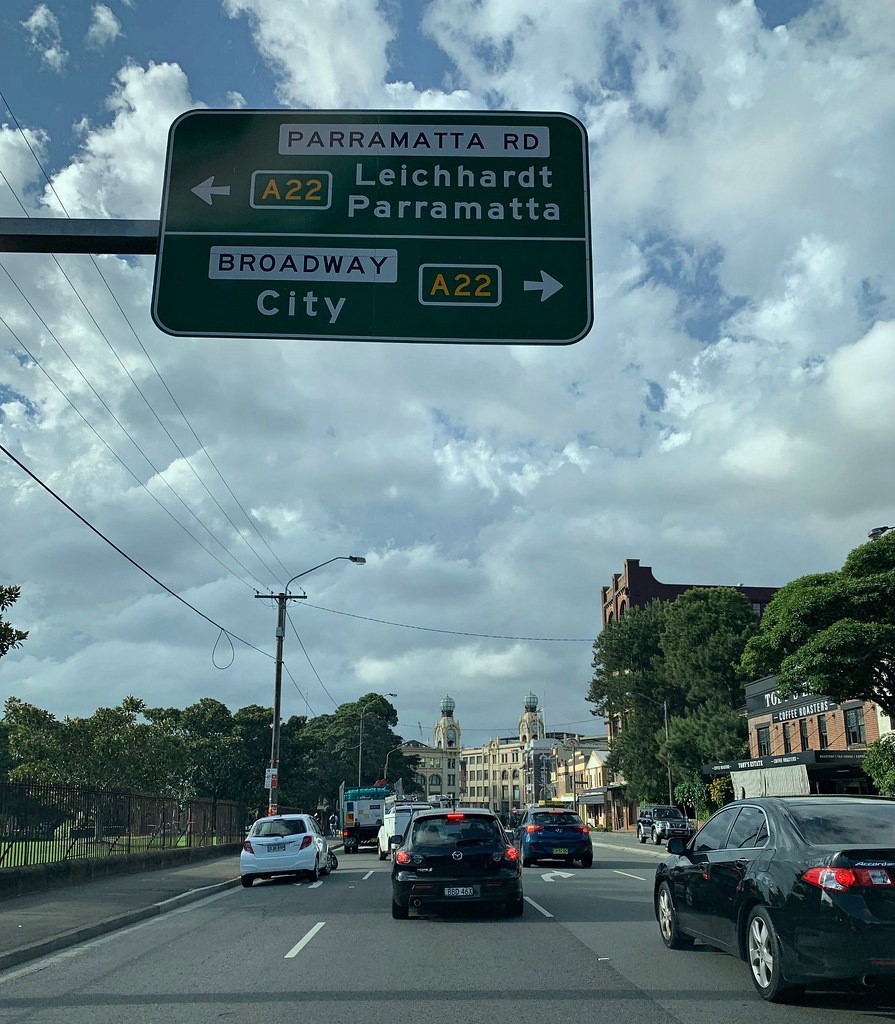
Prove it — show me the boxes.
[334,778,405,855]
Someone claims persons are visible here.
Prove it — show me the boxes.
[329,812,338,837]
[313,812,321,826]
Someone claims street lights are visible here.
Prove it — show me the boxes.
[357,691,398,788]
[625,690,674,806]
[267,553,368,814]
[552,743,576,810]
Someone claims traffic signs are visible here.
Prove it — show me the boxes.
[149,105,595,346]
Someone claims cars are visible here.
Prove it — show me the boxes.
[653,792,895,1005]
[238,812,334,888]
[515,807,594,869]
[387,807,524,920]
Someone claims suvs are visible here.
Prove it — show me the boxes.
[636,804,698,846]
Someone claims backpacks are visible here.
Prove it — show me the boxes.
[330,815,336,824]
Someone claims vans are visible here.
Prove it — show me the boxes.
[376,794,459,864]
